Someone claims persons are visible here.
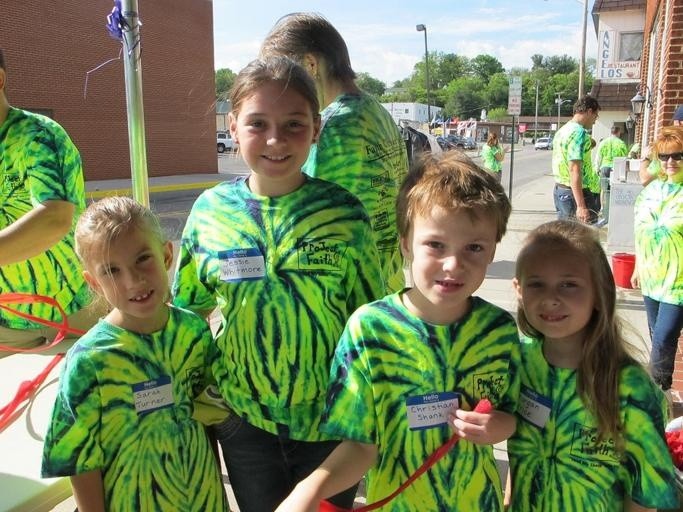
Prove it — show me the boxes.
[584,133,601,225]
[259,10,410,296]
[40,196,222,512]
[0,51,95,330]
[630,126,683,390]
[275,149,520,512]
[480,132,510,184]
[503,221,683,512]
[170,55,382,511]
[639,104,683,187]
[595,126,628,224]
[552,96,602,225]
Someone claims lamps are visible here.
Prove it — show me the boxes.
[626,111,640,129]
[629,83,653,116]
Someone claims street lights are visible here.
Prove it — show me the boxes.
[554,93,571,130]
[415,20,432,137]
[556,98,571,130]
[417,23,431,121]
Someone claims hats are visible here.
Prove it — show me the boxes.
[672,106,683,120]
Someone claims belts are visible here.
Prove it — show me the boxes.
[555,182,571,190]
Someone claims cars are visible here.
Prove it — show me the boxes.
[438,134,469,153]
[533,136,552,150]
[448,132,476,151]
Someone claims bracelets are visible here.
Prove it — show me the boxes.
[643,157,652,163]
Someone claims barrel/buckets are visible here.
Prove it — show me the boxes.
[611,252,638,289]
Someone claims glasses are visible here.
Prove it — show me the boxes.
[658,152,683,162]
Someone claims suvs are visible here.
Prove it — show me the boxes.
[216,132,235,153]
